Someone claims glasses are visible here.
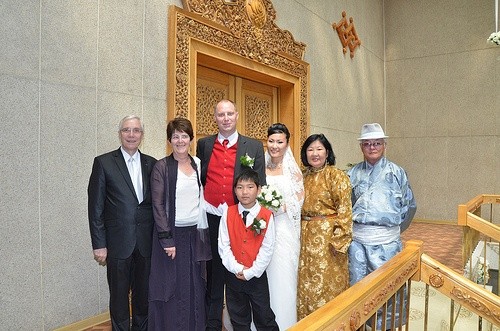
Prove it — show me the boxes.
[364,142,384,148]
[121,128,143,134]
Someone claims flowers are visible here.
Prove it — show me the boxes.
[252,218,266,235]
[257,184,284,213]
[487,0,500,46]
[238,153,255,169]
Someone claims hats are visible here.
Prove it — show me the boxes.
[357,123,389,141]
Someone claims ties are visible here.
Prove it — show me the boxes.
[223,139,229,149]
[129,157,138,193]
[242,211,249,225]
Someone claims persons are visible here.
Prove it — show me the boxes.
[346,123,417,331]
[222,122,305,331]
[196,98,267,331]
[218,169,279,331]
[297,134,352,324]
[87,115,159,331]
[149,117,212,331]
